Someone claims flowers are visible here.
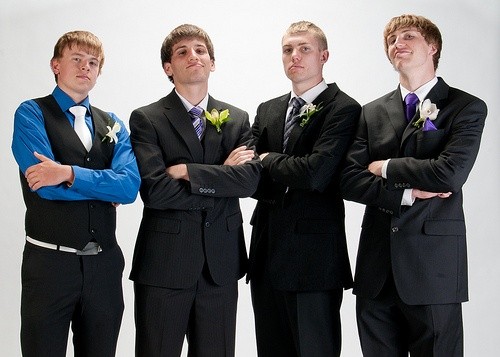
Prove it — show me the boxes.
[101,119,122,144]
[204,108,233,133]
[412,99,440,128]
[299,101,324,127]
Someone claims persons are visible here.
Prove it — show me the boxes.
[128,24,263,357]
[340,14,487,357]
[12,31,141,357]
[246,22,363,357]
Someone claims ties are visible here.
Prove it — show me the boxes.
[69,106,92,152]
[404,93,419,123]
[282,97,306,155]
[189,107,204,143]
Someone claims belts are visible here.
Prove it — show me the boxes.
[26,236,103,255]
